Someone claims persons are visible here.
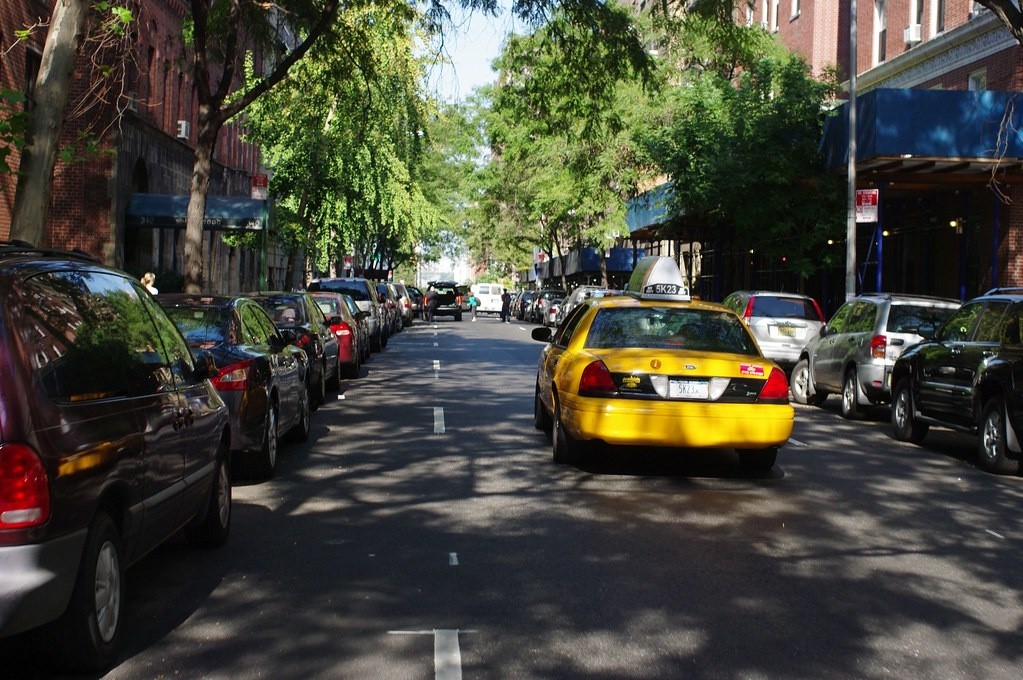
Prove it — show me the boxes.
[467,292,477,322]
[501,289,511,321]
[425,290,440,322]
[140,272,158,296]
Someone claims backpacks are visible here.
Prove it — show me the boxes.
[474,297,481,307]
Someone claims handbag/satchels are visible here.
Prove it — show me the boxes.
[426,290,438,301]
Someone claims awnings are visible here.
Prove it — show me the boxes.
[127,192,277,290]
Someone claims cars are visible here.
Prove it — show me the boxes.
[722,291,826,364]
[456,285,472,312]
[505,285,625,329]
[307,292,372,380]
[378,283,424,337]
[155,296,312,484]
[528,296,795,477]
[308,278,382,355]
[246,291,342,412]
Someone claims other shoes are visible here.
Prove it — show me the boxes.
[508,318,510,322]
[501,319,506,322]
[472,317,476,322]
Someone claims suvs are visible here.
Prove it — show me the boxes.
[0,240,232,676]
[424,281,463,322]
[789,293,964,420]
[891,287,1023,474]
